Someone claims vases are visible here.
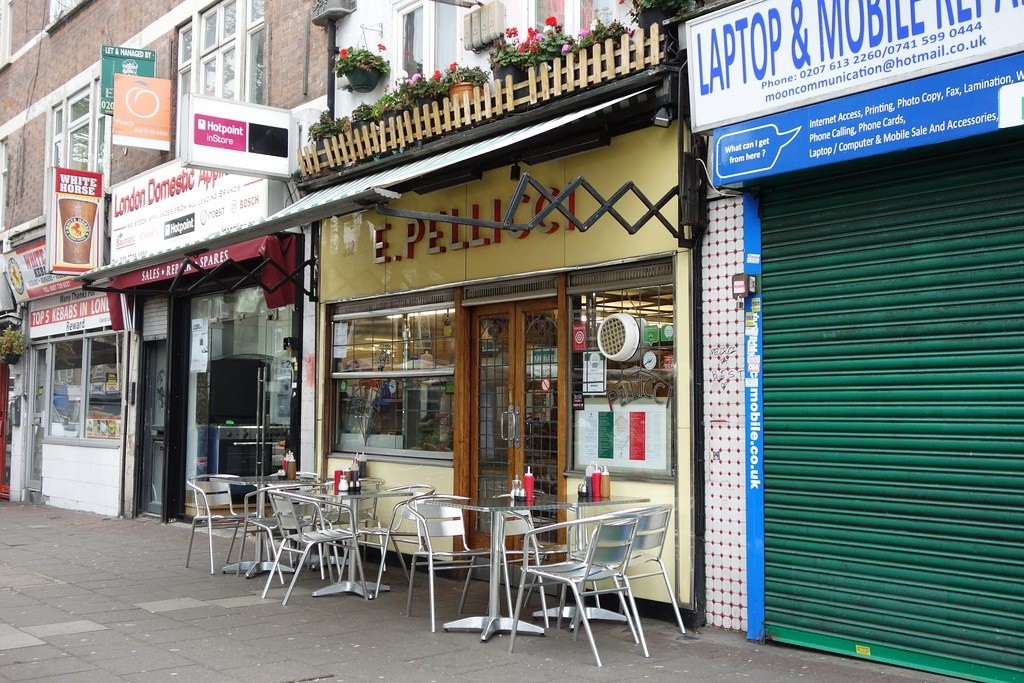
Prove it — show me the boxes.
[492,59,527,101]
[417,93,445,115]
[537,53,570,89]
[449,81,484,105]
[344,68,383,94]
[583,40,618,77]
[637,5,676,37]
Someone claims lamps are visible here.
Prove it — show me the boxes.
[510,157,521,181]
[651,107,675,129]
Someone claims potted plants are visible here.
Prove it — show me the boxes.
[0,326,27,365]
[349,99,376,139]
[375,81,412,129]
[305,110,347,163]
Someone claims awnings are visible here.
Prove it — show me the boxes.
[72,86,678,297]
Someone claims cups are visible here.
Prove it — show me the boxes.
[59,198,98,266]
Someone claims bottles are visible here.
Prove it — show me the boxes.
[283,450,296,479]
[351,450,367,482]
[524,466,534,501]
[334,464,343,491]
[344,464,351,485]
[585,461,610,497]
[512,475,522,496]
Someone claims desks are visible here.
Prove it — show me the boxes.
[303,486,413,602]
[210,473,322,580]
[431,483,654,646]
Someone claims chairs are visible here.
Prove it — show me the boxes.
[399,487,495,636]
[356,464,442,599]
[505,510,648,670]
[265,466,320,568]
[560,492,689,644]
[307,471,387,569]
[488,480,560,634]
[235,480,327,585]
[183,469,256,575]
[258,489,369,608]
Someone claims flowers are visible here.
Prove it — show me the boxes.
[401,0,691,96]
[332,40,392,77]
